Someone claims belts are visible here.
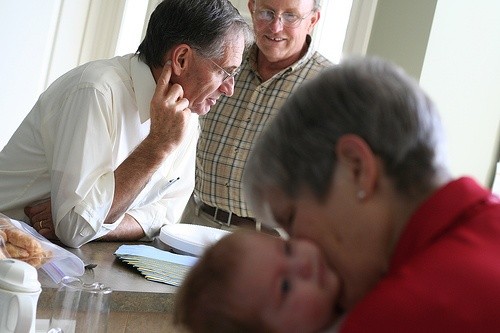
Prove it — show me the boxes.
[191,193,278,235]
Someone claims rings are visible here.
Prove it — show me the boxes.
[40,221,44,229]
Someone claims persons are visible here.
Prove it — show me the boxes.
[1,0,248,249]
[178,0,341,241]
[170,56,500,333]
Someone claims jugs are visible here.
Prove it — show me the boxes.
[0,259,42,333]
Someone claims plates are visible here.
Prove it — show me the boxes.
[159,224,234,258]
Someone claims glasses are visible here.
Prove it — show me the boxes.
[252,9,314,27]
[191,47,242,82]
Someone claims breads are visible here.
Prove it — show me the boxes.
[0,218,52,268]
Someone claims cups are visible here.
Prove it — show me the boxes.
[48,276,112,333]
